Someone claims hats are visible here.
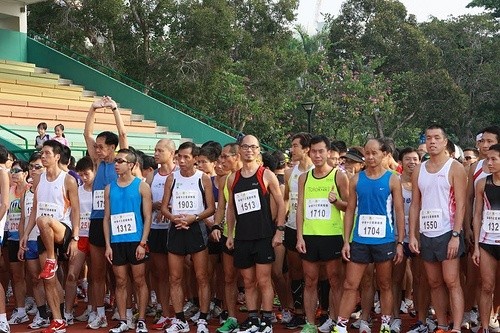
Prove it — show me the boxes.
[340,148,365,162]
[454,144,460,160]
[476,133,483,141]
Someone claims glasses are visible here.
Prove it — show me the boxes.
[10,168,23,174]
[93,144,103,149]
[221,153,236,159]
[28,163,42,170]
[241,144,258,150]
[465,156,471,160]
[113,158,130,163]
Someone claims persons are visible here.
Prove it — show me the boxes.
[225,135,286,333]
[16,140,80,332]
[472,143,500,333]
[330,138,404,333]
[2,127,500,333]
[408,123,467,333]
[35,122,69,146]
[161,140,216,332]
[294,135,350,333]
[83,96,129,332]
[103,149,153,333]
[0,144,11,333]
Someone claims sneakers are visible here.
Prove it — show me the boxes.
[0,279,500,333]
[39,258,58,279]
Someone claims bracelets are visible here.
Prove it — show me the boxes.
[211,225,220,231]
[329,199,337,204]
[112,106,118,111]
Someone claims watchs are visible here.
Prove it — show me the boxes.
[275,226,285,232]
[194,213,200,221]
[71,235,80,241]
[140,242,147,248]
[451,231,461,237]
[397,241,404,246]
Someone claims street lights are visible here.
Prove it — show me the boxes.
[300,102,316,134]
